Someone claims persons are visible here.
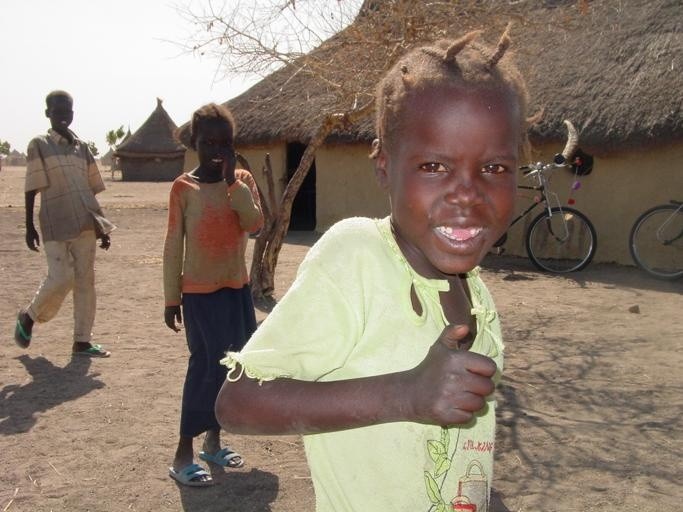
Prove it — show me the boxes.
[213,29,530,512]
[15,90,117,358]
[162,104,265,486]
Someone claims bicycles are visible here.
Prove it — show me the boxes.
[491,147,600,275]
[625,197,682,281]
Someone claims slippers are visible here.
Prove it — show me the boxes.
[70,343,112,360]
[167,463,215,489]
[13,306,32,350]
[198,446,246,469]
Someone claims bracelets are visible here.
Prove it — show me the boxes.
[225,176,235,183]
